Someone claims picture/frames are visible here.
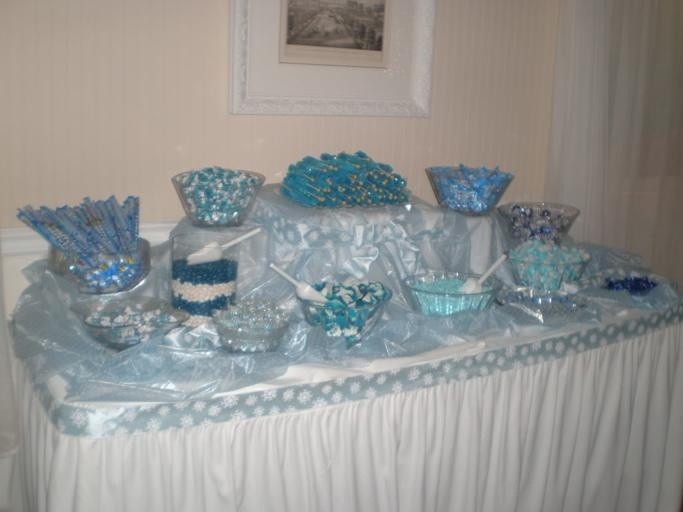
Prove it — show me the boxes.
[228,1,436,119]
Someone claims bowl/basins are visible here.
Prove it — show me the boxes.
[210,304,296,358]
[70,294,190,352]
[169,170,267,230]
[46,243,152,291]
[397,275,503,319]
[496,202,583,244]
[293,279,394,356]
[506,249,595,292]
[424,167,516,217]
